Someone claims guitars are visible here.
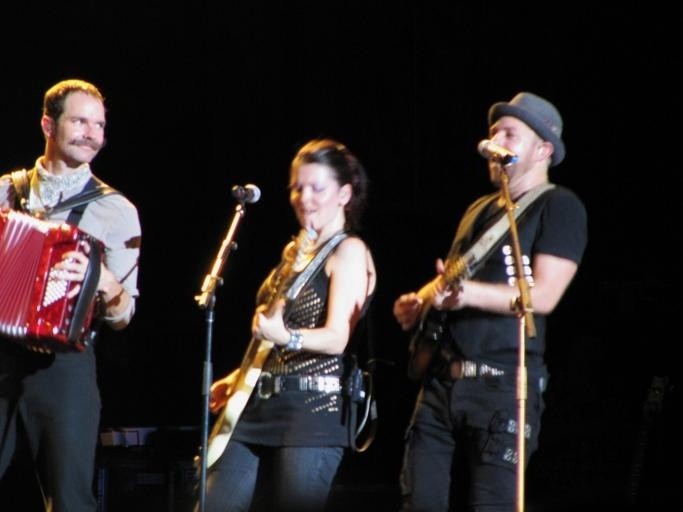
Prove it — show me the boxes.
[194,228,316,476]
[406,253,473,381]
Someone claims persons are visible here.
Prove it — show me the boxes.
[1,79,142,511]
[192,137,378,511]
[391,91,588,512]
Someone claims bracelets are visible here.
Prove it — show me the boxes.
[104,287,125,308]
[288,331,301,352]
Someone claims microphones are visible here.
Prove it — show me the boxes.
[232,184,261,203]
[477,140,513,165]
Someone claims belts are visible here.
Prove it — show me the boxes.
[257,372,343,402]
[442,360,505,379]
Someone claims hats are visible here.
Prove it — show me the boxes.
[488,92,566,167]
